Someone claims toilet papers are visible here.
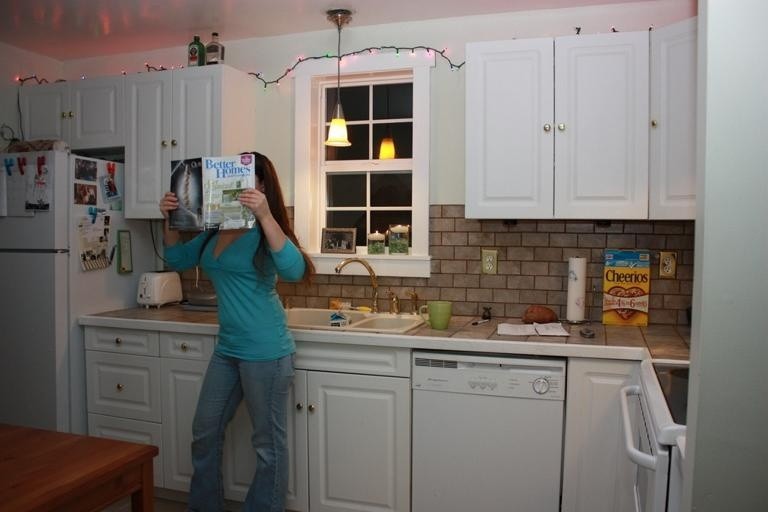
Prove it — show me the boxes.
[566,258,587,322]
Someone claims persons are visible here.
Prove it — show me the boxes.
[159,151,317,510]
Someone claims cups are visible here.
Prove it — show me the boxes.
[368,233,385,254]
[389,224,409,255]
[419,301,452,330]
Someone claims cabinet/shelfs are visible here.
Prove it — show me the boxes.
[559,355,640,510]
[23,76,125,150]
[465,30,650,221]
[161,332,223,494]
[124,64,256,220]
[85,326,164,489]
[223,340,411,512]
[649,15,697,219]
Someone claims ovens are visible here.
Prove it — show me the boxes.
[614,382,675,512]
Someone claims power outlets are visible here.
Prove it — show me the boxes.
[659,252,676,280]
[482,250,498,274]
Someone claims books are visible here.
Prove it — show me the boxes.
[170,156,256,231]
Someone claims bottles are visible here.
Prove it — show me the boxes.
[188,35,205,66]
[205,32,224,64]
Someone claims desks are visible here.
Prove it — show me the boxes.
[0,423,159,511]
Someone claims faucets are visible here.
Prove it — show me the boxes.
[336,258,378,313]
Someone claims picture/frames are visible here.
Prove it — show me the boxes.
[320,228,356,254]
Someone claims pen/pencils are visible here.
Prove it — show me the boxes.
[472,319,489,325]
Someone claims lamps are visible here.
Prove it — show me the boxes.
[380,86,396,159]
[326,9,354,148]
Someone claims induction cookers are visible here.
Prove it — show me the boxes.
[653,360,689,427]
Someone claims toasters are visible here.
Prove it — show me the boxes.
[137,271,183,309]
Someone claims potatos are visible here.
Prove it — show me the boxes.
[522,305,558,323]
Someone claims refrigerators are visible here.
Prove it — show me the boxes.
[0,151,157,435]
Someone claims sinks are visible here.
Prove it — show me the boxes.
[350,312,429,334]
[285,308,368,329]
